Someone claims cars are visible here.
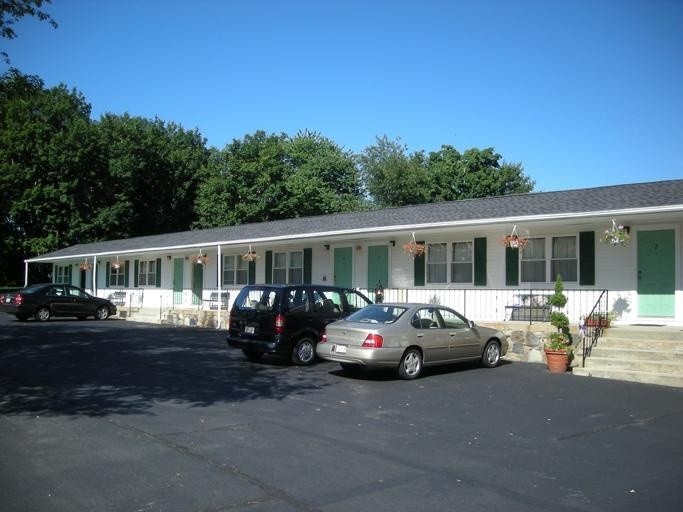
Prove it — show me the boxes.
[0,283,115,322]
[314,303,510,379]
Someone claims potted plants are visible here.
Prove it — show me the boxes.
[584,311,615,327]
[543,270,573,373]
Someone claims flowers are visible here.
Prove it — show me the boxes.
[79,263,91,272]
[600,216,630,247]
[400,242,426,257]
[500,234,528,249]
[188,256,208,267]
[110,260,123,271]
[239,253,259,263]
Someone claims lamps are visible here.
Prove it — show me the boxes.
[323,244,330,251]
[166,255,172,261]
[388,240,396,248]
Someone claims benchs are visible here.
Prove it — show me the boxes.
[197,291,230,310]
[107,291,127,302]
[503,293,551,322]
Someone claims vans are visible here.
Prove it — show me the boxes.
[224,283,374,364]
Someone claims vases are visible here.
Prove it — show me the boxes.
[509,240,517,248]
[196,260,203,264]
[114,265,119,269]
[611,236,620,245]
